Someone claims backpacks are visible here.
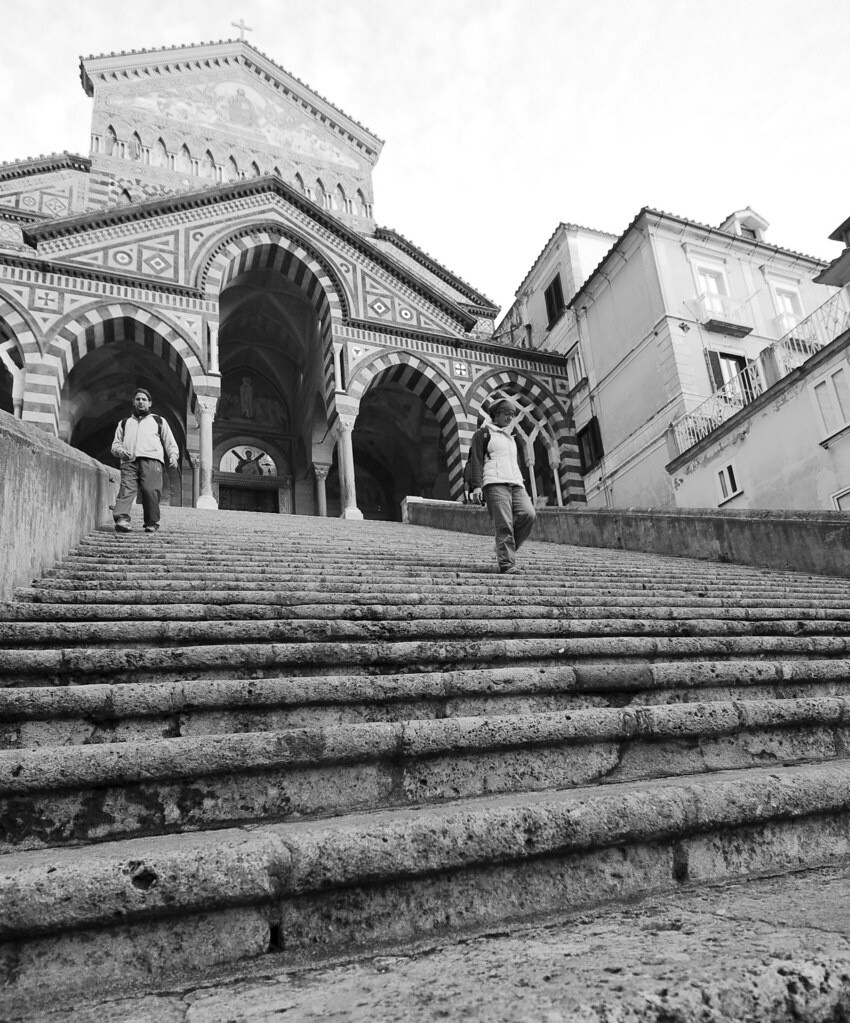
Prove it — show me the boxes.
[463,427,491,490]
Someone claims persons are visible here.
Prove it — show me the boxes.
[109,389,179,532]
[463,400,536,574]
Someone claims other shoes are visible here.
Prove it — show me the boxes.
[115,520,134,532]
[145,526,156,532]
[505,566,521,575]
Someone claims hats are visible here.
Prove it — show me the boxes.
[489,398,515,416]
